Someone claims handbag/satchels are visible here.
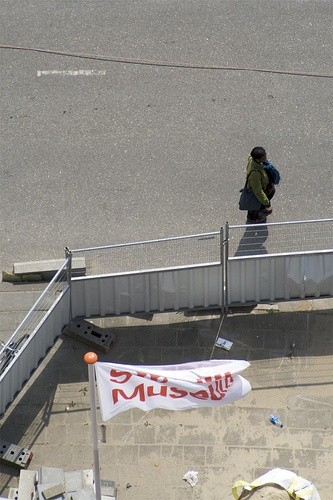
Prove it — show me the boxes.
[239,169,263,211]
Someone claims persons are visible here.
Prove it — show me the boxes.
[245,147,275,222]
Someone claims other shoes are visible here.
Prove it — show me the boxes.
[248,216,266,223]
[261,207,272,213]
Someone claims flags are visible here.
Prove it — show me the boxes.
[95,359,253,425]
